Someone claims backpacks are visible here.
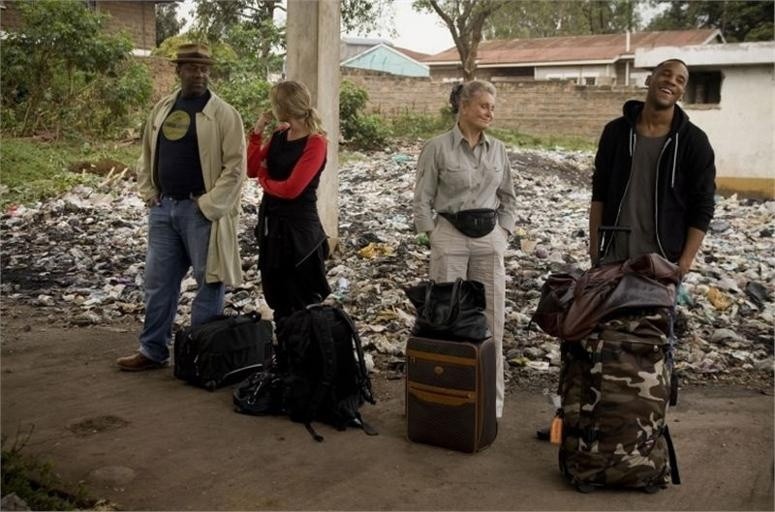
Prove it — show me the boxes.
[233,301,379,441]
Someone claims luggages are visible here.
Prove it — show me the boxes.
[405,339,498,454]
[527,252,681,494]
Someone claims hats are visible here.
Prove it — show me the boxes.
[164,42,218,64]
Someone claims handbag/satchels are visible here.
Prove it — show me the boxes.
[173,309,273,393]
[438,208,496,238]
[404,278,486,339]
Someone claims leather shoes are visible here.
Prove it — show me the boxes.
[116,352,171,372]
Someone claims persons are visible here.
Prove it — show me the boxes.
[411,79,517,421]
[116,42,246,370]
[536,59,716,443]
[246,80,329,367]
